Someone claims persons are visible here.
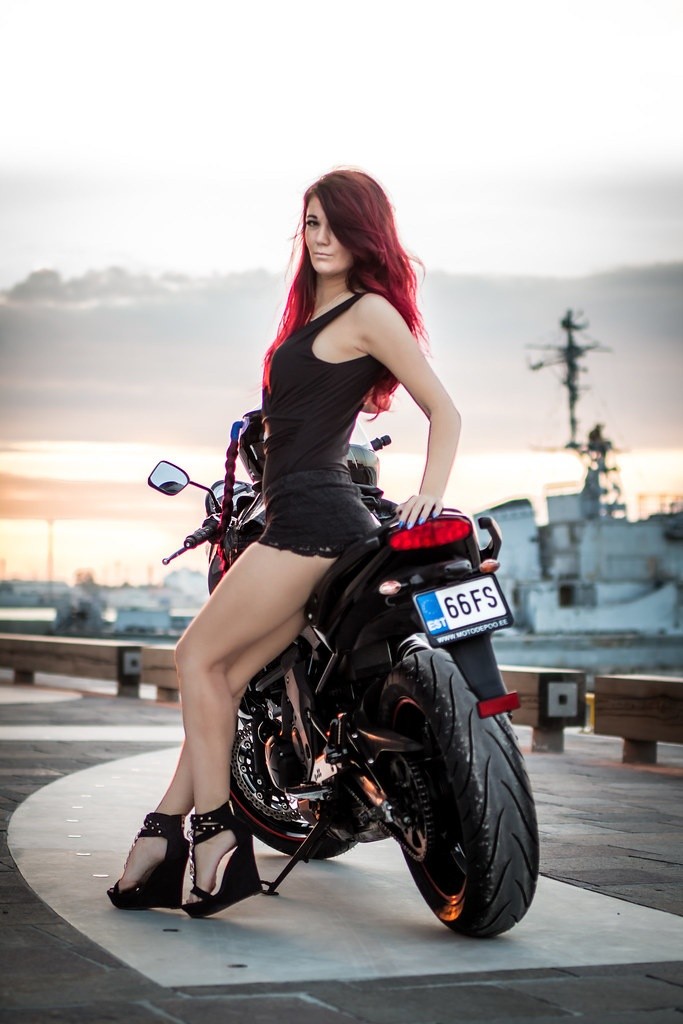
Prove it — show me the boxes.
[107,169,462,919]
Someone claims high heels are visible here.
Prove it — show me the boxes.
[108,802,263,920]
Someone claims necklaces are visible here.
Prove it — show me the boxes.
[310,288,349,319]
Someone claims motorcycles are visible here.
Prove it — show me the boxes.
[148,411,541,940]
